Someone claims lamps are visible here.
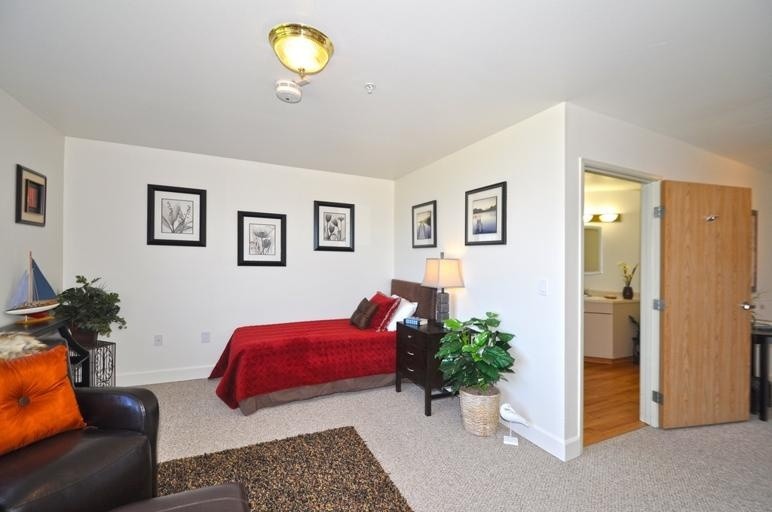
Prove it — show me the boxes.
[420,252,467,323]
[584,214,593,223]
[268,23,335,79]
[599,214,619,223]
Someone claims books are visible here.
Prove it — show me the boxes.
[403,316,429,326]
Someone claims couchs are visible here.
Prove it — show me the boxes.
[53,275,127,346]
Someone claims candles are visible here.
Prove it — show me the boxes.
[433,312,516,437]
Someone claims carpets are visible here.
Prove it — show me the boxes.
[123,425,413,512]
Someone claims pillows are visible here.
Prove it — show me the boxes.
[1,345,87,455]
[350,291,418,332]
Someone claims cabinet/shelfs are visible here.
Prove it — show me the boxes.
[584,290,640,365]
[0,315,90,387]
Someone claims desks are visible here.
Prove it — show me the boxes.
[750,328,772,422]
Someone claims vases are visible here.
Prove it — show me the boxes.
[623,287,634,299]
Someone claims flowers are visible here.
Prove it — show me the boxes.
[623,263,639,287]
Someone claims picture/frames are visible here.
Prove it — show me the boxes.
[312,198,356,253]
[16,162,48,228]
[461,180,507,247]
[144,183,209,249]
[409,199,439,249]
[235,209,290,268]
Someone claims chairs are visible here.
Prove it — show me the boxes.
[0,337,160,506]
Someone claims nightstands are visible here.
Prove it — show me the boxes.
[395,320,472,417]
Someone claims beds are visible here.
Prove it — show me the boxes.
[235,278,436,409]
